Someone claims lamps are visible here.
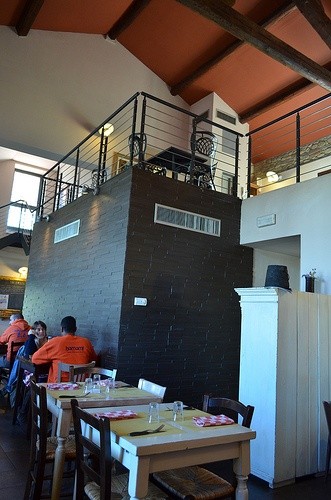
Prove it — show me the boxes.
[18,267,28,278]
[134,297,148,306]
[98,123,114,184]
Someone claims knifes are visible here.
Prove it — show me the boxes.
[165,407,192,411]
[129,430,167,435]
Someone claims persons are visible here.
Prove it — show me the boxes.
[0,313,32,367]
[16,320,54,427]
[31,316,97,383]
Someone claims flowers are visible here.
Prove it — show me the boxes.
[301,267,319,279]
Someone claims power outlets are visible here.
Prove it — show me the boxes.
[46,215,50,221]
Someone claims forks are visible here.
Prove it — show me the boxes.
[59,392,91,398]
[128,424,165,436]
[167,407,195,410]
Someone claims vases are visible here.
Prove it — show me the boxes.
[304,277,316,293]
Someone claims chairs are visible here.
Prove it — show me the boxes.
[120,130,218,192]
[0,341,255,500]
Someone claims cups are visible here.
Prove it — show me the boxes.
[172,401,184,422]
[147,402,159,424]
[84,375,115,396]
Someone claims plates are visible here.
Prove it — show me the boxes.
[49,383,77,388]
[96,380,129,387]
[76,382,99,387]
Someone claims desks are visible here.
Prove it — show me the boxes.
[133,146,208,181]
[35,381,162,500]
[72,402,256,500]
[11,355,52,435]
